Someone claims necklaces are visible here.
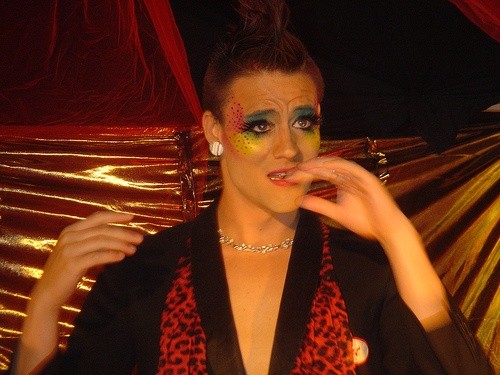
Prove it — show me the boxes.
[217,227,293,255]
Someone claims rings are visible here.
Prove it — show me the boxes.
[330,167,336,183]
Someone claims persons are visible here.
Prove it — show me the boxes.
[5,26,496,375]
